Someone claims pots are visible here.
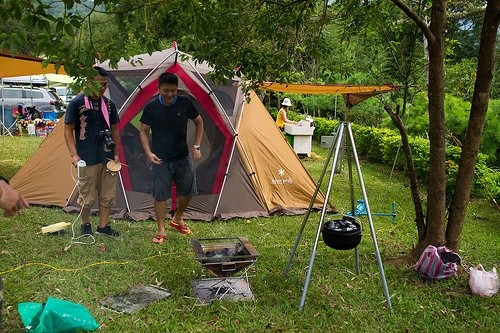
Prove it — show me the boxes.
[322,214,363,250]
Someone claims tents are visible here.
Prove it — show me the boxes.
[2,48,392,222]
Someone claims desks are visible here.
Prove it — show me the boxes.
[286,133,312,157]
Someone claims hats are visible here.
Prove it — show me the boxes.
[282,98,293,106]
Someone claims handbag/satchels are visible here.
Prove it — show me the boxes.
[415,244,458,280]
[469,264,499,298]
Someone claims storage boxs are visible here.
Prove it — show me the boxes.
[284,123,313,136]
[35,111,60,137]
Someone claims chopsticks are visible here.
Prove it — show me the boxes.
[107,158,129,167]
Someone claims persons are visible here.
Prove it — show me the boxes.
[140,73,204,244]
[276,98,296,132]
[0,176,30,218]
[64,66,120,238]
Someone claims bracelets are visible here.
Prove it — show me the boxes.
[193,144,200,150]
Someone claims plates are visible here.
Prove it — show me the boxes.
[106,160,122,171]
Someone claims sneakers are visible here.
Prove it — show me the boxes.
[80,222,93,238]
[96,224,120,238]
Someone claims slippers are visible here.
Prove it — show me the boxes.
[152,235,166,244]
[169,220,191,234]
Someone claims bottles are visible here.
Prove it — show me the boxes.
[76,159,86,179]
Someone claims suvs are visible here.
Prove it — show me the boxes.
[0,84,63,111]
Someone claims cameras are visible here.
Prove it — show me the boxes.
[98,130,116,150]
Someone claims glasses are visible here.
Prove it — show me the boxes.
[100,82,110,88]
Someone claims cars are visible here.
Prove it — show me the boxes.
[52,86,78,108]
